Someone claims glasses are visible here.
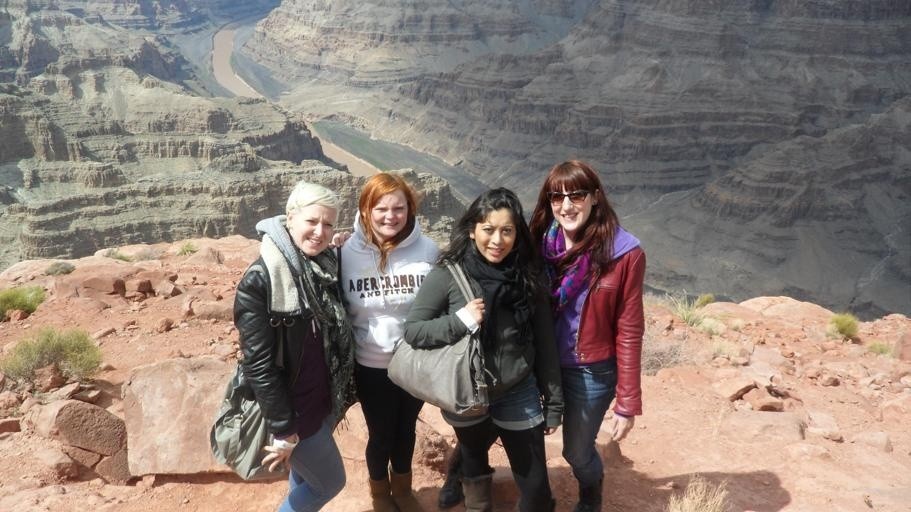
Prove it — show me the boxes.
[546,191,593,205]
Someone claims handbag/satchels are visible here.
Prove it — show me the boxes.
[209,367,287,480]
[386,327,489,417]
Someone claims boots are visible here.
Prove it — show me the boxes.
[438,440,496,512]
[573,476,604,511]
[369,465,424,512]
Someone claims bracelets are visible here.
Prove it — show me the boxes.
[273,433,299,450]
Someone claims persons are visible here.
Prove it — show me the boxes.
[401,186,567,512]
[232,183,352,512]
[437,159,647,512]
[330,171,442,512]
